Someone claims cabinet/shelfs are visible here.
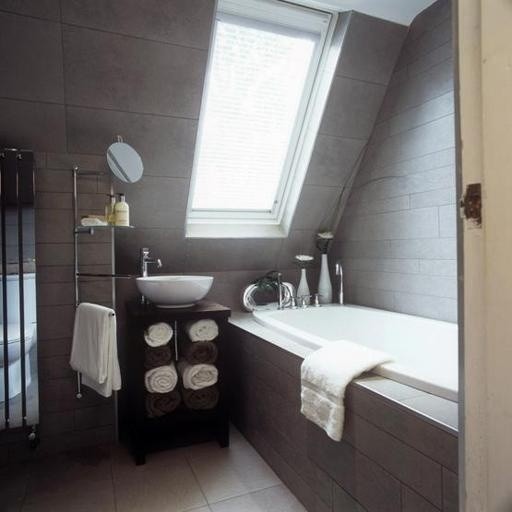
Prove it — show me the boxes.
[123,300,231,466]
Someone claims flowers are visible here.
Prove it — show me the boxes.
[294,254,313,269]
[316,231,334,253]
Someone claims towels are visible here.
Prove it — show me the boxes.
[296,341,395,442]
[140,316,219,421]
[69,302,122,399]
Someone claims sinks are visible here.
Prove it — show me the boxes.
[136,274,214,309]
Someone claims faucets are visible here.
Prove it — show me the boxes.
[140,247,162,303]
[334,262,348,304]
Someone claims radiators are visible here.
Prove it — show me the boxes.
[0,149,43,443]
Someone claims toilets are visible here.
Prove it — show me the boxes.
[0,273,38,403]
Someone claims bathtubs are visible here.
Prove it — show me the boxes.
[251,300,459,406]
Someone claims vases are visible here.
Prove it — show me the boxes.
[296,269,312,306]
[318,254,332,305]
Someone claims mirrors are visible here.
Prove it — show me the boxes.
[105,140,145,183]
